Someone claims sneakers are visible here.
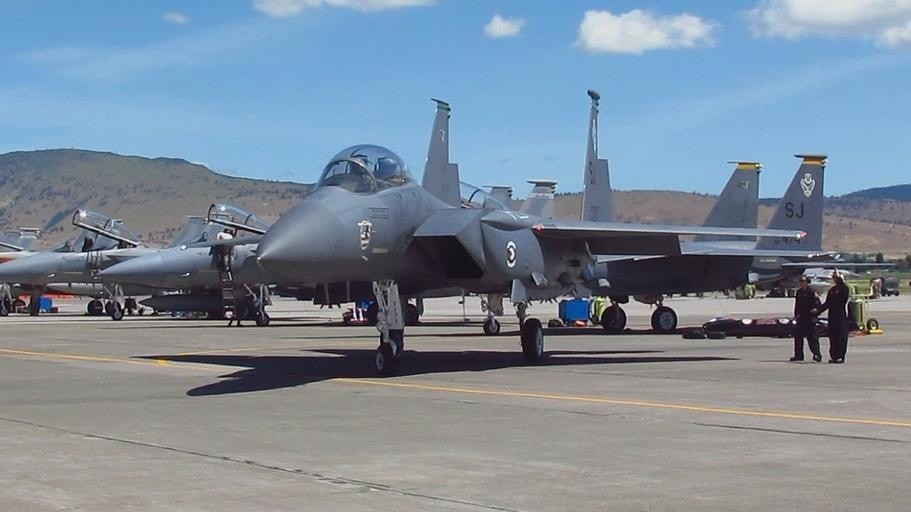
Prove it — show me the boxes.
[813,354,822,362]
[829,358,844,363]
[790,357,804,361]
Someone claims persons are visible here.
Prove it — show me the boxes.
[812,267,850,364]
[30,289,42,316]
[324,154,376,193]
[372,156,411,193]
[790,276,823,362]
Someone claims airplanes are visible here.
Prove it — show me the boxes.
[0,88,900,380]
